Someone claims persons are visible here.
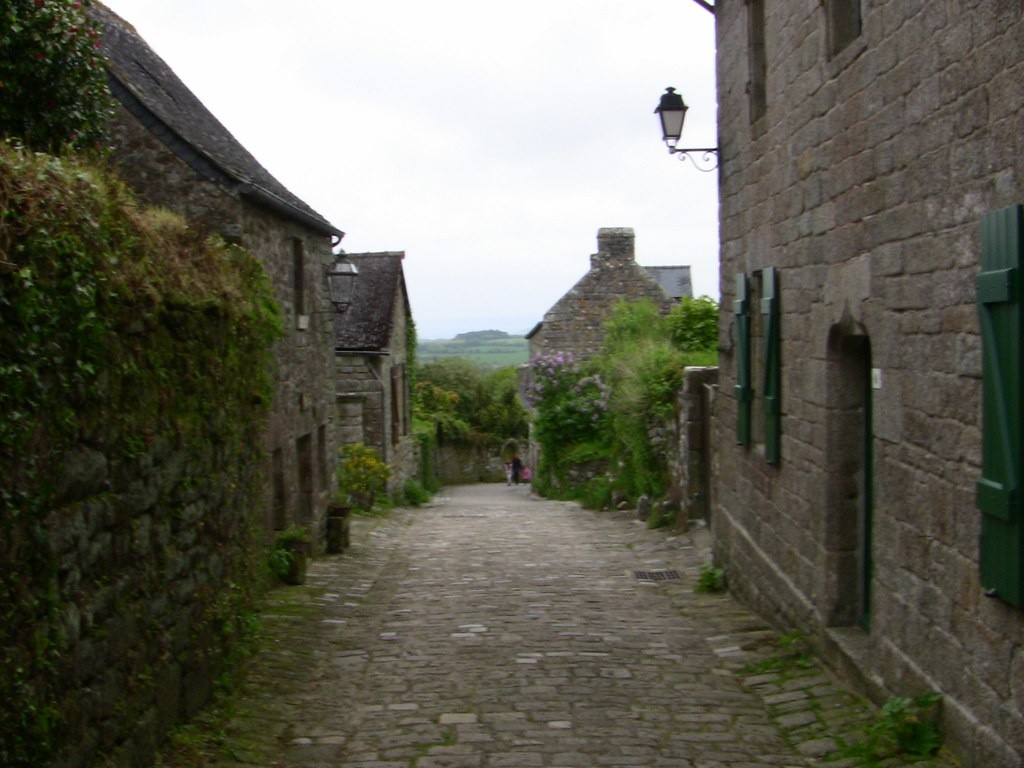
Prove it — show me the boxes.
[505,454,530,486]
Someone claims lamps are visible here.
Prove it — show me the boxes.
[654,87,718,173]
[311,250,359,315]
